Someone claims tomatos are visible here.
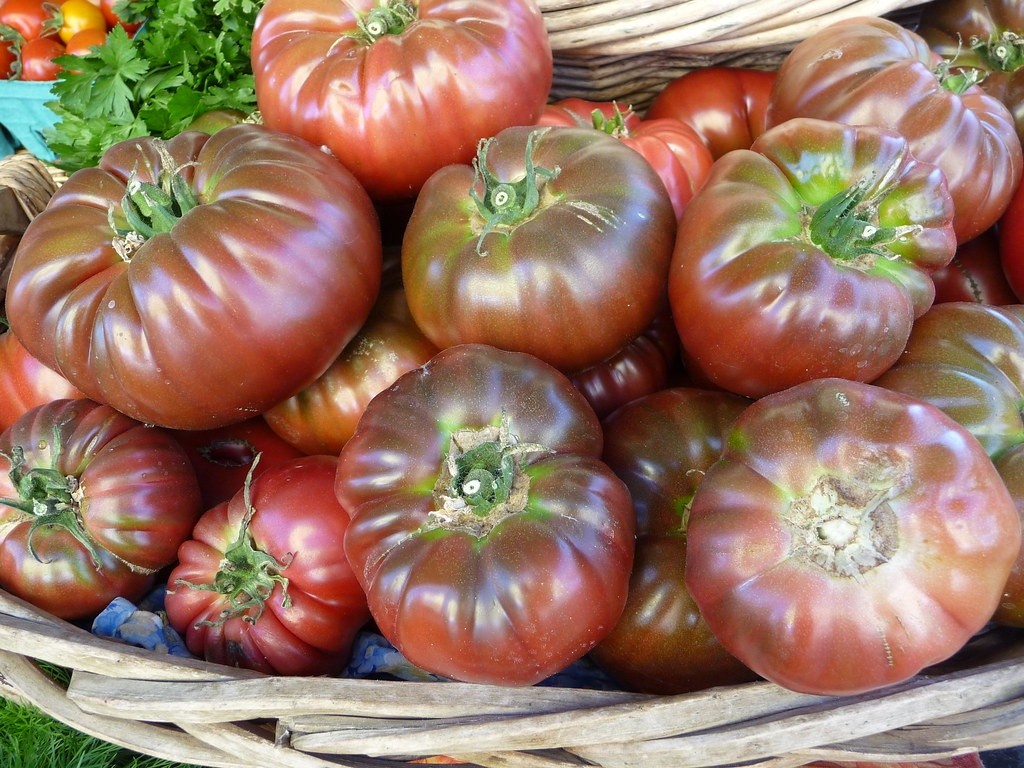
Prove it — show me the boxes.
[0,0,1024,698]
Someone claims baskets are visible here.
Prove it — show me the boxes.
[1,147,1024,768]
[539,0,928,111]
